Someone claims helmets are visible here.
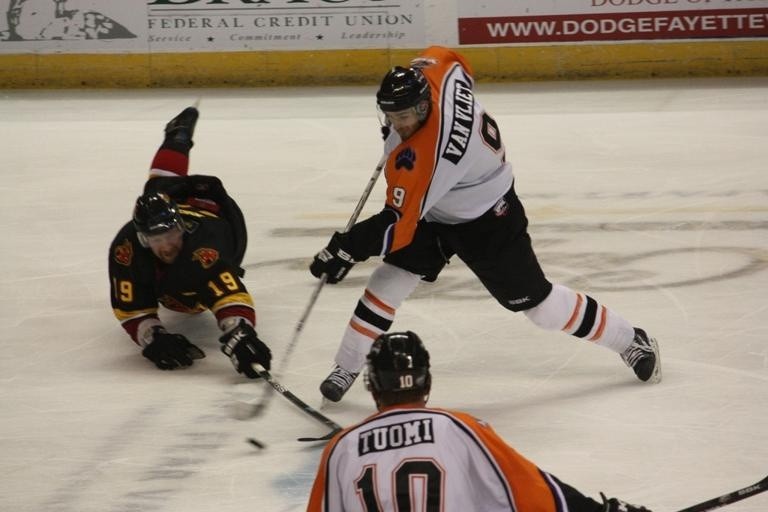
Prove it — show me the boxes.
[132,191,186,247]
[363,330,430,404]
[375,65,431,129]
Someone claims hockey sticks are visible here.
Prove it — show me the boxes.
[252,364,344,443]
[226,154,386,422]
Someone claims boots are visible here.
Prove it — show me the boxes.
[165,107,199,148]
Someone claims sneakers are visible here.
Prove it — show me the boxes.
[320,365,359,401]
[619,327,654,381]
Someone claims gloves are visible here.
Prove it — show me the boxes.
[310,231,356,284]
[603,498,654,511]
[141,332,205,371]
[218,319,271,379]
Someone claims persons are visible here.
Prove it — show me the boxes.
[108,105,274,381]
[310,46,657,404]
[304,328,655,512]
